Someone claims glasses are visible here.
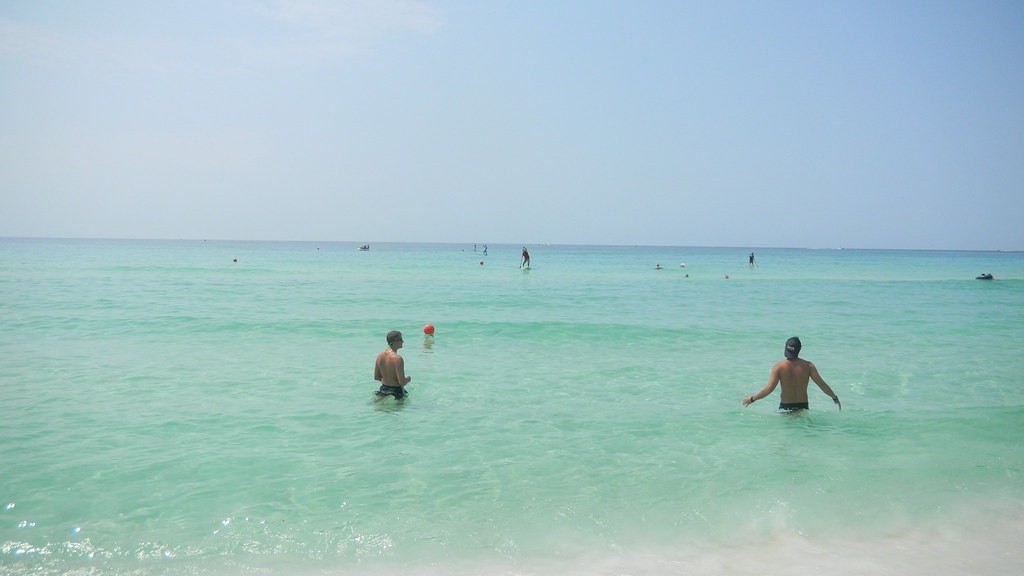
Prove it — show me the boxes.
[393,338,403,342]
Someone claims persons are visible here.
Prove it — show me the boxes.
[748,252,755,268]
[743,336,842,413]
[482,244,487,256]
[519,247,530,269]
[655,263,663,269]
[374,330,411,396]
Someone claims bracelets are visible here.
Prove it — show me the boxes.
[750,396,755,402]
[832,396,839,403]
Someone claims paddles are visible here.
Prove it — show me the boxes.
[753,258,759,267]
[519,247,524,268]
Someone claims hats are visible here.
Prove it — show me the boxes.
[784,337,801,359]
